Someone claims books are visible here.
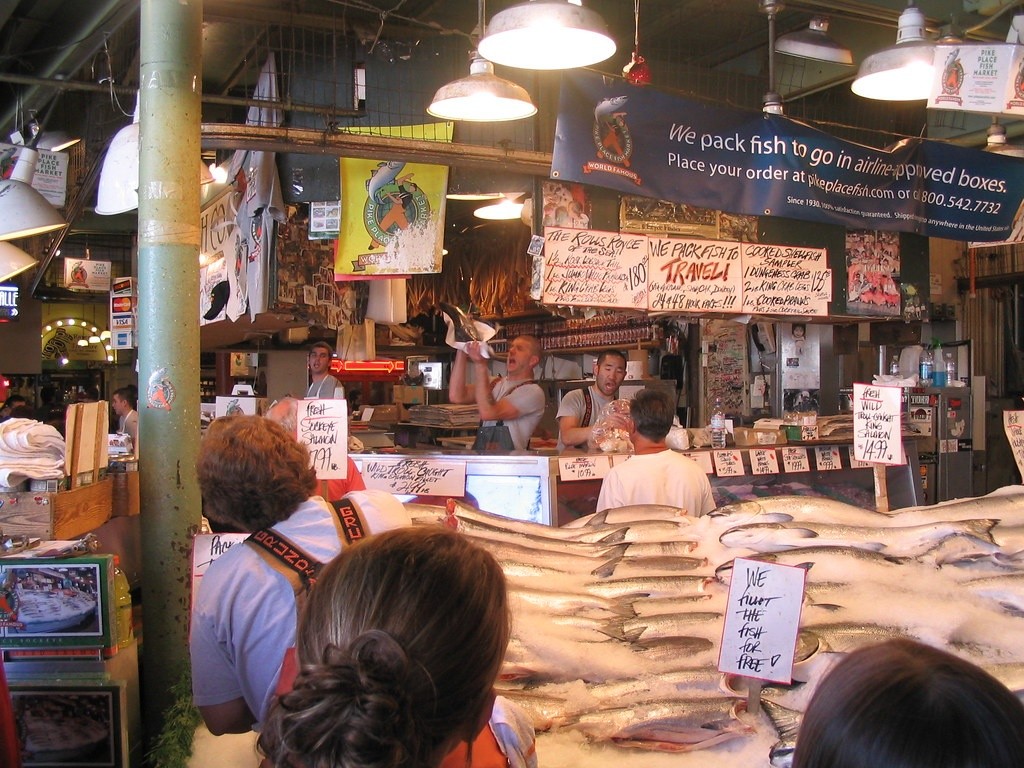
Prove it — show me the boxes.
[0,558,110,686]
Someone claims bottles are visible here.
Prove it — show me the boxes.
[890,355,899,375]
[919,343,933,387]
[57,462,68,492]
[711,398,726,449]
[933,349,947,387]
[63,386,85,401]
[945,353,955,386]
[114,555,134,649]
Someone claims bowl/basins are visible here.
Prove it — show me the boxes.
[779,425,802,441]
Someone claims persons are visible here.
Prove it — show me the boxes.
[556,348,633,450]
[596,388,716,517]
[263,396,366,503]
[0,396,66,441]
[303,341,344,400]
[110,386,142,461]
[789,638,1024,768]
[187,414,538,767]
[257,525,513,768]
[449,334,546,451]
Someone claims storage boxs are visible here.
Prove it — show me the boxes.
[393,384,424,404]
[0,475,114,542]
[0,137,87,208]
[107,471,140,517]
[925,42,1024,115]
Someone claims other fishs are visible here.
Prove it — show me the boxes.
[365,161,406,205]
[399,482,1024,768]
[436,299,495,360]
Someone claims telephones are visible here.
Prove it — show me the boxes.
[750,321,776,355]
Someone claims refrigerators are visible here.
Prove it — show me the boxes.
[937,393,973,502]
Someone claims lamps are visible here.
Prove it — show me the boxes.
[0,87,69,243]
[25,108,82,153]
[0,240,40,284]
[979,115,1024,157]
[89,303,101,343]
[478,0,618,70]
[849,0,940,100]
[425,23,538,122]
[100,304,111,338]
[772,14,856,66]
[78,303,88,346]
[94,88,216,215]
